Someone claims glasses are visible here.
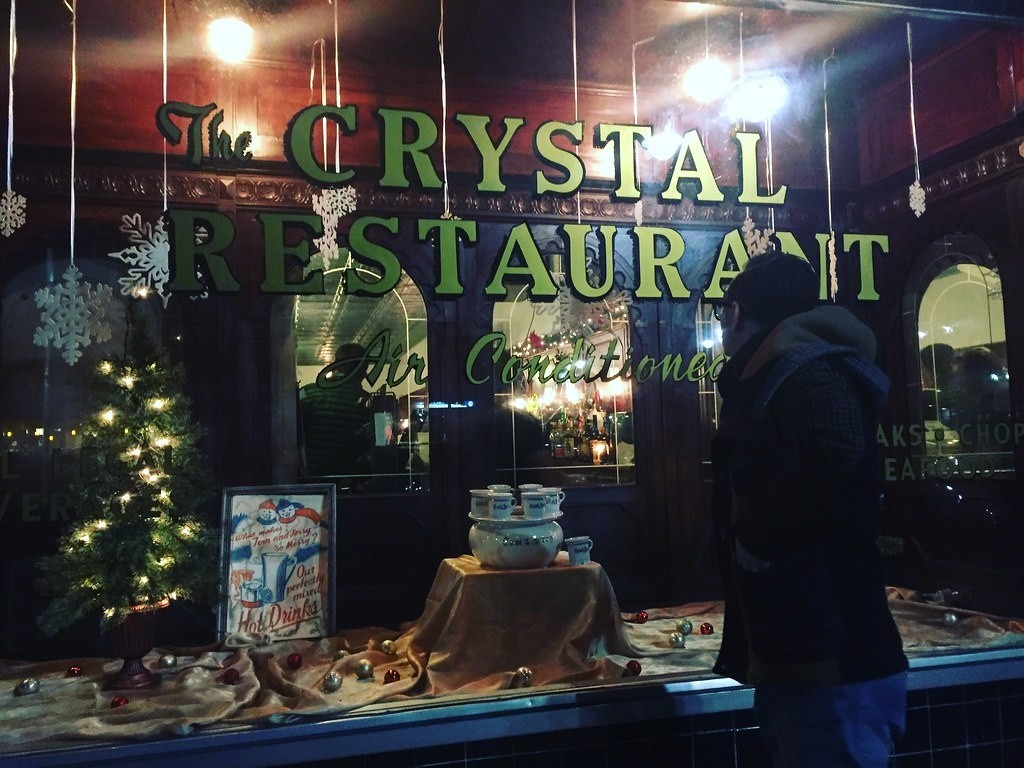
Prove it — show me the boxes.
[710,299,732,320]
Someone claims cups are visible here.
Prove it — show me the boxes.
[469,489,494,519]
[564,536,593,565]
[537,487,565,515]
[487,492,517,520]
[487,484,515,496]
[518,483,543,496]
[521,491,552,520]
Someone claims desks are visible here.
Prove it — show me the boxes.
[436,549,604,662]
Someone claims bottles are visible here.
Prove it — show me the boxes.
[536,405,632,465]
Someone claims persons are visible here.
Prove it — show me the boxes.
[492,346,553,469]
[921,342,1014,471]
[706,249,910,768]
[298,343,409,477]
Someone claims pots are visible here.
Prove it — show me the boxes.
[468,506,565,569]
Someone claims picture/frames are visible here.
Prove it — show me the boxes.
[215,482,339,650]
